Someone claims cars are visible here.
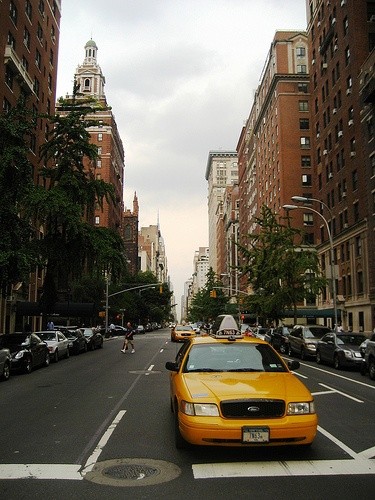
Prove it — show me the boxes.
[166,314,318,449]
[108,325,129,335]
[0,345,11,381]
[239,322,274,343]
[32,331,73,365]
[171,323,195,343]
[359,331,375,380]
[75,327,104,351]
[135,322,161,334]
[168,323,200,334]
[316,332,373,370]
[271,324,299,354]
[0,332,49,375]
[54,328,88,356]
[288,325,333,360]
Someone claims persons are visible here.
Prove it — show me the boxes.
[110,324,115,337]
[120,322,135,354]
[336,323,345,333]
[47,320,54,331]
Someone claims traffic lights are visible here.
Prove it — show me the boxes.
[210,290,214,297]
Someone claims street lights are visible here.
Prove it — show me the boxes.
[138,286,156,327]
[221,273,241,328]
[282,194,340,331]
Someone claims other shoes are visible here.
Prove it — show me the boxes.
[131,351,135,353]
[121,350,125,353]
